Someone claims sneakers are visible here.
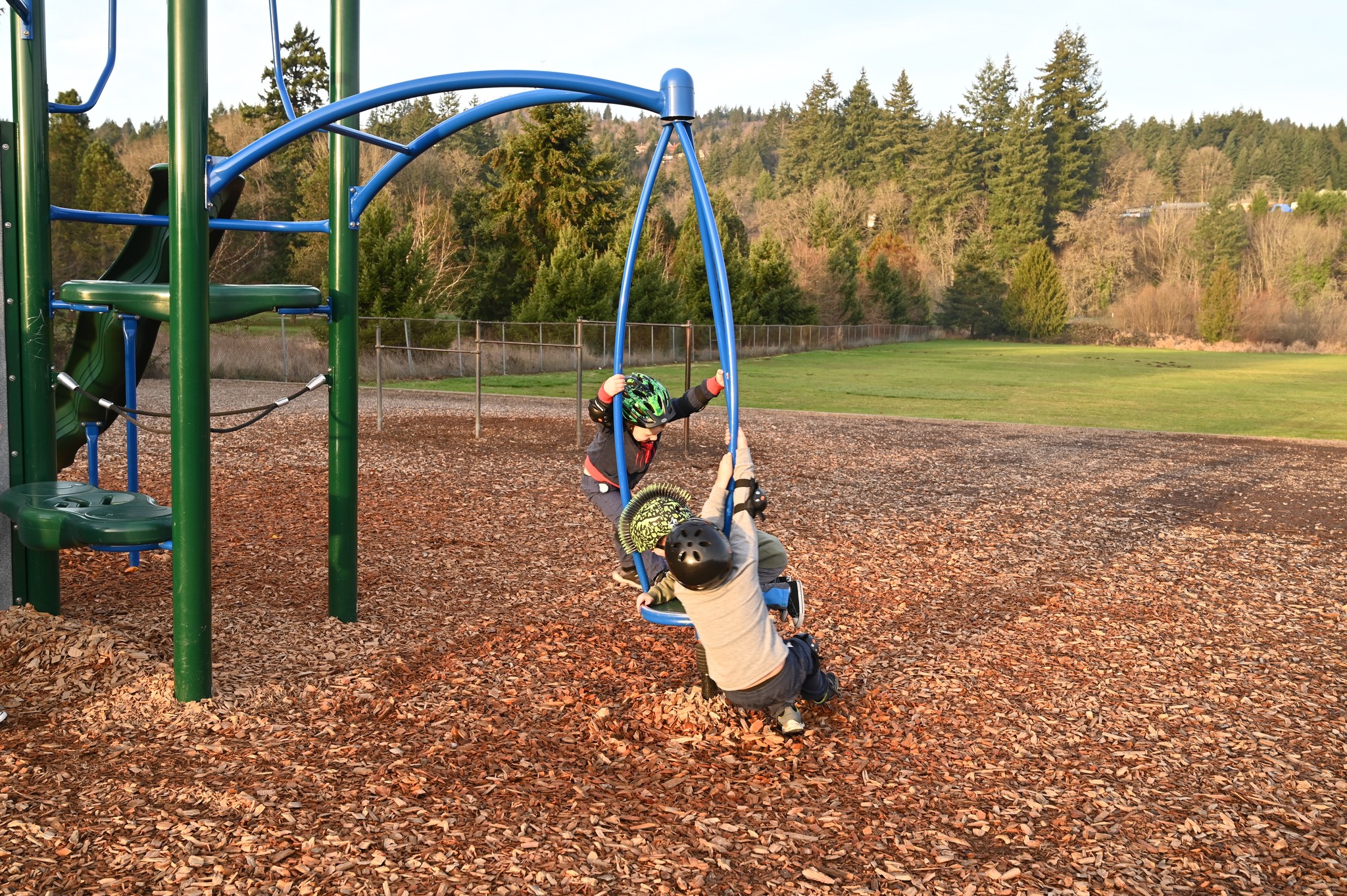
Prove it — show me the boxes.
[787,579,805,628]
[612,568,655,589]
[778,576,791,623]
[778,705,805,735]
[812,671,839,705]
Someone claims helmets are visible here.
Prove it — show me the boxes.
[622,372,676,429]
[664,517,733,592]
[617,482,691,555]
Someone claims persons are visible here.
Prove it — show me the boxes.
[663,427,838,733]
[579,369,729,591]
[618,482,804,628]
